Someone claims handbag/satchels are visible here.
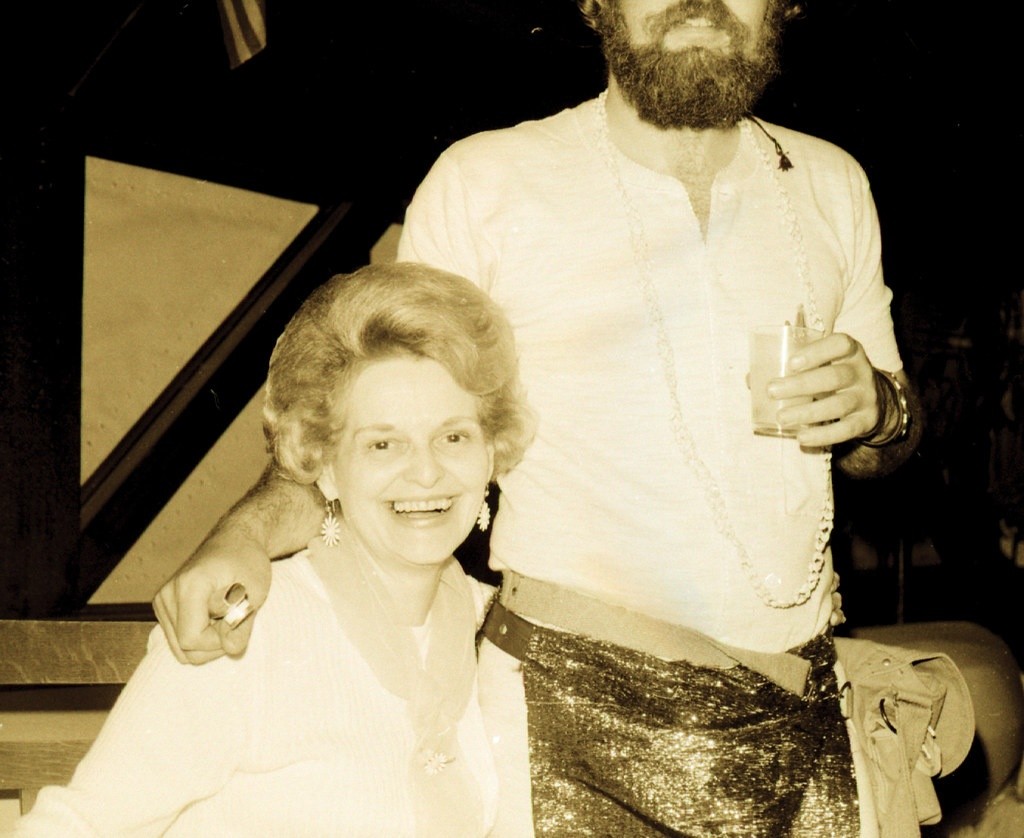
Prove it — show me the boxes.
[497,570,975,838]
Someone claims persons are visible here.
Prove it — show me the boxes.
[0,259,536,838]
[147,1,921,838]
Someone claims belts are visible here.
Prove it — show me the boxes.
[483,600,542,666]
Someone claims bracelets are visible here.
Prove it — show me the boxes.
[858,369,910,449]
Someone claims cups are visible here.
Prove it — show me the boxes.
[751,324,825,438]
